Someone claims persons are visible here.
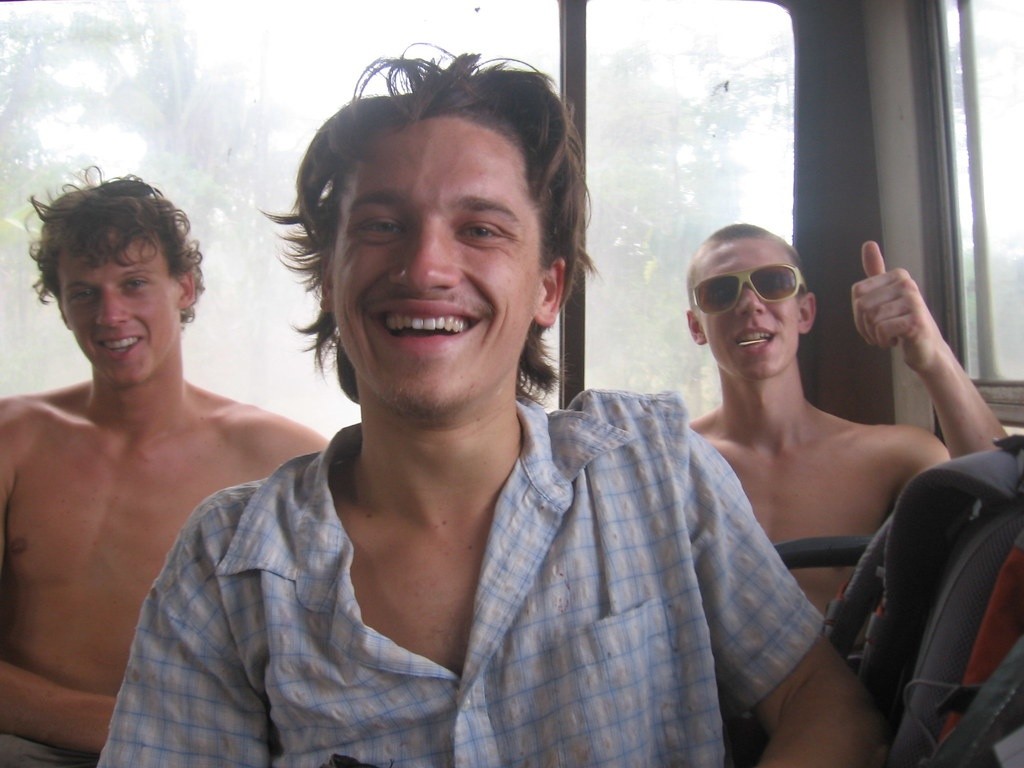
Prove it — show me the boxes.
[685,225,1011,617]
[0,163,329,768]
[94,37,892,767]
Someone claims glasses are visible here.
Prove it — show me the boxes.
[692,263,808,315]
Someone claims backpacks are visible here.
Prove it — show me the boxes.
[824,434,1024,768]
